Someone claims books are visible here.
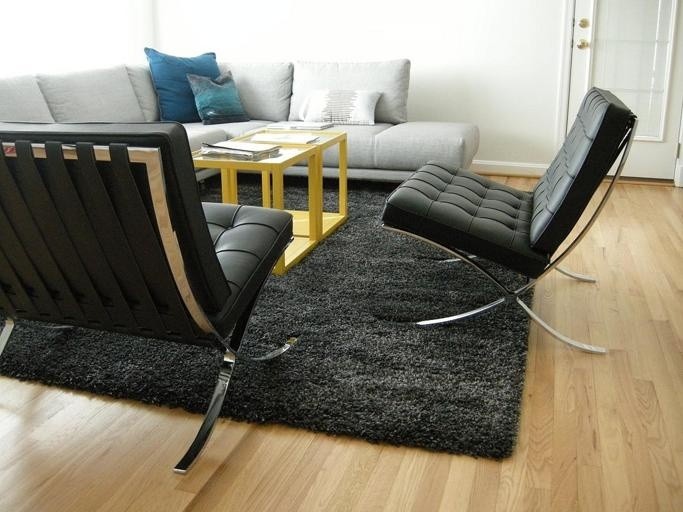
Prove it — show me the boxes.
[201,121,334,162]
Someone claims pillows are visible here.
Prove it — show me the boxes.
[145,46,246,124]
[303,89,382,125]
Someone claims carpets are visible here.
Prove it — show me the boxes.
[0,179,535,462]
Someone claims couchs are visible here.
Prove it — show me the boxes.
[0,59,481,182]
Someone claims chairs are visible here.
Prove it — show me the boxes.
[379,86,637,357]
[1,122,299,472]
